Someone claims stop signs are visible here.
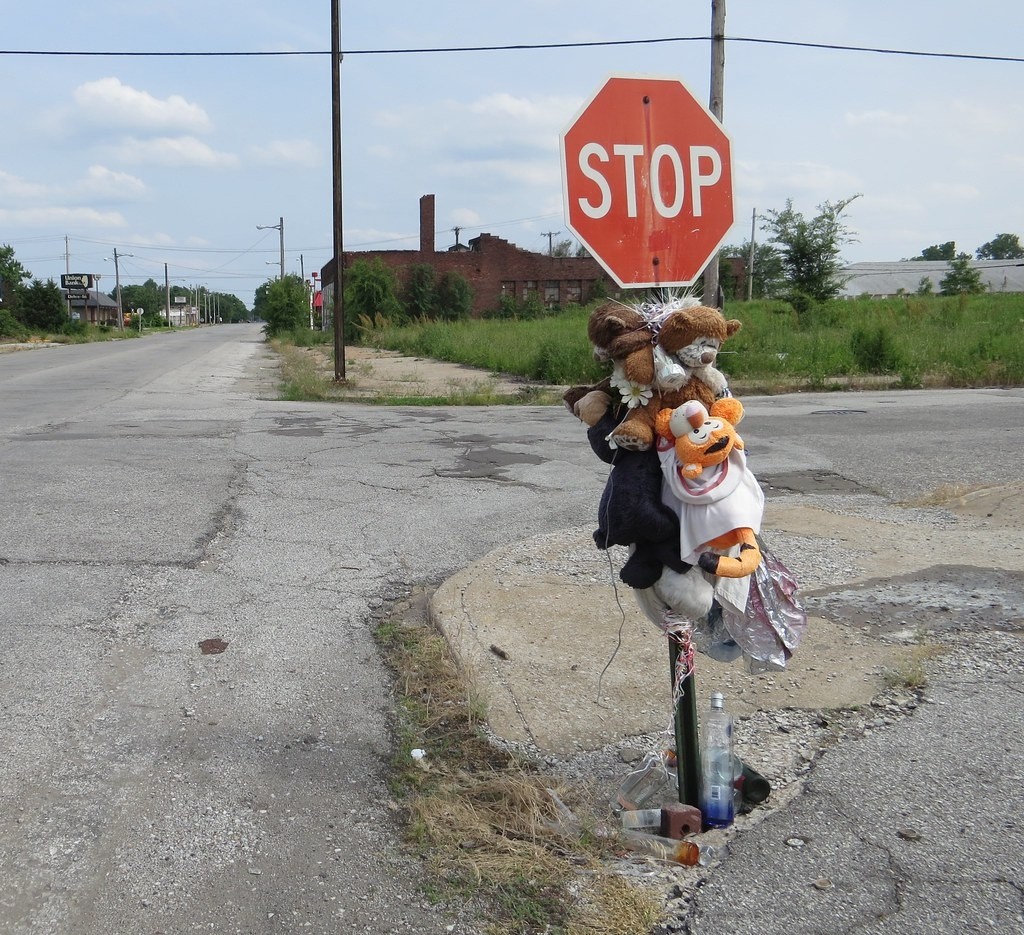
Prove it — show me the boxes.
[561,79,733,291]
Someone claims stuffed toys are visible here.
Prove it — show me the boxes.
[564,298,764,634]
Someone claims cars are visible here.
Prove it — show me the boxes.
[100,319,117,327]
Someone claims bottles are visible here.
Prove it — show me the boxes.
[545,820,588,846]
[606,746,678,812]
[698,845,714,867]
[597,828,698,865]
[702,692,771,825]
[617,808,661,829]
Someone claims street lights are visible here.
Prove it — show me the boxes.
[257,217,286,283]
[102,247,135,331]
[167,279,185,325]
